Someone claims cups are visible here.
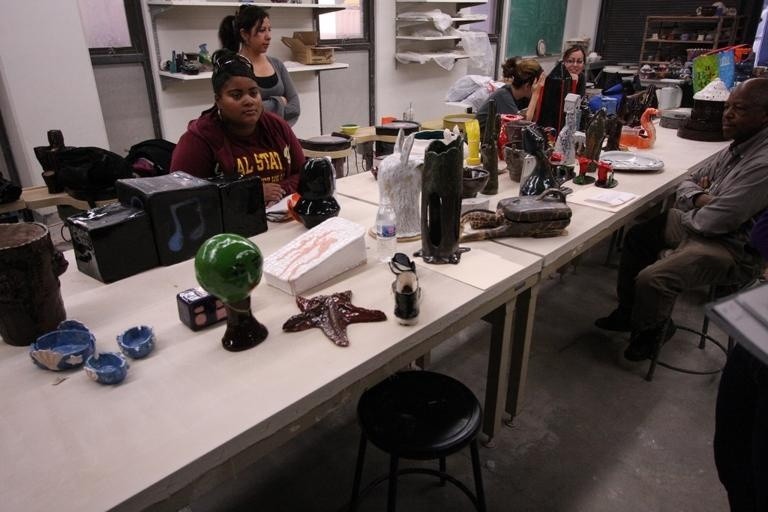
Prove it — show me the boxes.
[393,272,424,325]
[504,142,523,182]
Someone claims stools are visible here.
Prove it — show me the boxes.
[349,369,485,512]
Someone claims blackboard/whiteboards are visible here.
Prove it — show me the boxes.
[498,1,569,68]
[593,0,765,64]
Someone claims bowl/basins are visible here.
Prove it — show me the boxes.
[462,167,490,199]
[339,123,359,135]
[442,115,476,132]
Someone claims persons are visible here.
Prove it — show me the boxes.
[474,56,547,140]
[537,45,588,130]
[169,48,307,209]
[217,4,301,129]
[593,77,768,362]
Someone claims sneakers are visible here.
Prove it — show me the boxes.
[622,317,677,361]
[593,305,634,334]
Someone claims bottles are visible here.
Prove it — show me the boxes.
[481,97,500,195]
[374,197,396,265]
[181,50,201,74]
[465,120,482,166]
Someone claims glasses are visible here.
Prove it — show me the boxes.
[213,55,254,80]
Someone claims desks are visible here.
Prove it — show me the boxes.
[333,95,736,420]
[0,193,545,512]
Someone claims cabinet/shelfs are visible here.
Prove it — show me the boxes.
[392,0,488,70]
[141,0,349,141]
[634,15,737,88]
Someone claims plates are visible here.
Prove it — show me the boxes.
[600,150,663,174]
